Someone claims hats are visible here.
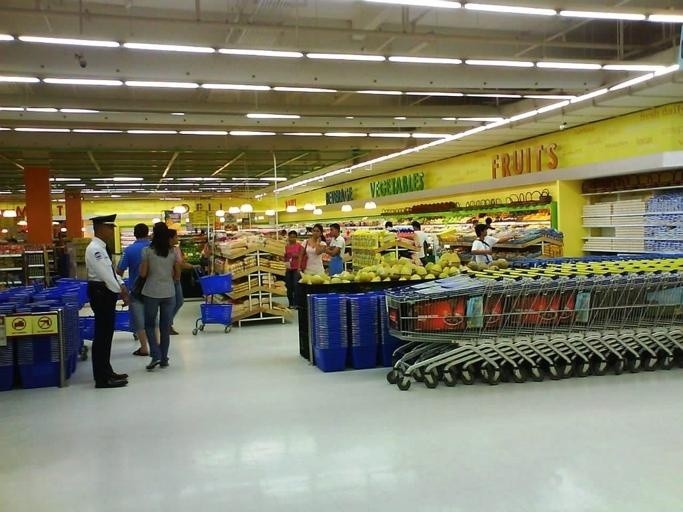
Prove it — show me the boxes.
[89,214,118,227]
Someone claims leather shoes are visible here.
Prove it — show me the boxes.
[113,373,129,379]
[112,380,129,387]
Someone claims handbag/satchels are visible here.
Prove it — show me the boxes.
[300,240,308,269]
[131,274,147,303]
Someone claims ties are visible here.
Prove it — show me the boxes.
[107,245,118,282]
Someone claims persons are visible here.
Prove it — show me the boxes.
[116,222,161,356]
[484,218,495,230]
[468,224,514,262]
[168,229,203,334]
[470,220,479,228]
[83,213,133,388]
[137,222,176,370]
[282,217,430,309]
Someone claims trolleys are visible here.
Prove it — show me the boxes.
[384,259,682,388]
[191,269,234,335]
[79,280,144,359]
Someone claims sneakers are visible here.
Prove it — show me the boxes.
[160,359,168,366]
[146,359,161,369]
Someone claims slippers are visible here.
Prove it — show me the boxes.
[133,348,148,356]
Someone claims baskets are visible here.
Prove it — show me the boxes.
[200,303,232,324]
[454,189,551,210]
[306,292,406,373]
[115,311,133,332]
[118,277,129,300]
[0,278,94,391]
[199,272,232,295]
[380,207,409,216]
